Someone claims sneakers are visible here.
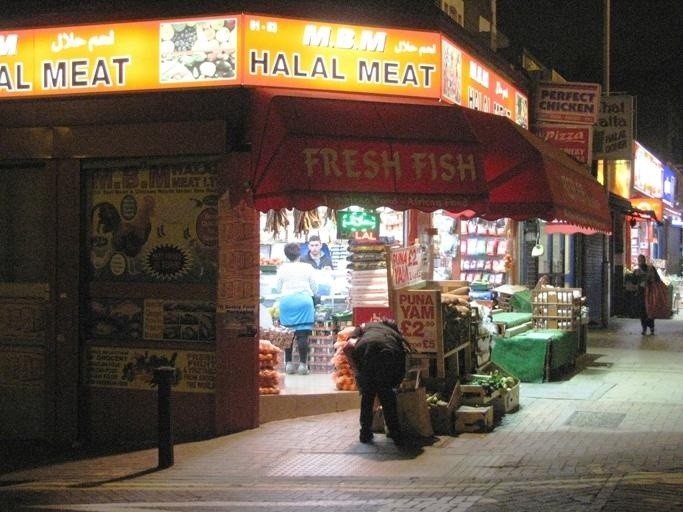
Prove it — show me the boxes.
[360,429,373,443]
[286,362,310,375]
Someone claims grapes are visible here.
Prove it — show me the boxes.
[172,26,197,52]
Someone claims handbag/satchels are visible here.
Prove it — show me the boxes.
[645,281,673,319]
[342,325,362,377]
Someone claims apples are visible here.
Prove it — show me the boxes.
[258,339,282,394]
[224,20,235,32]
[335,331,356,391]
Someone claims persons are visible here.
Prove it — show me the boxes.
[277,235,335,374]
[344,318,407,448]
[631,254,660,335]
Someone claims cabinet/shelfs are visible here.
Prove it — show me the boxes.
[530,285,584,351]
